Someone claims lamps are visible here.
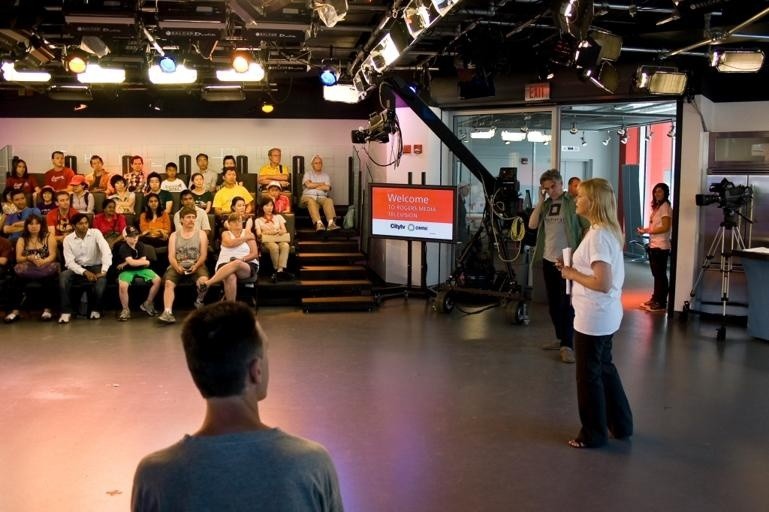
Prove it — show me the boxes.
[0,1,472,106]
[490,114,678,149]
[560,0,765,97]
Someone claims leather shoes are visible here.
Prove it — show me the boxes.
[281,271,294,280]
[561,346,575,363]
[271,272,277,281]
[541,336,561,350]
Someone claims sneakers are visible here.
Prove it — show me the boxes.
[193,297,204,309]
[315,220,326,233]
[5,301,176,324]
[198,279,207,293]
[646,302,666,312]
[327,220,341,231]
[641,295,653,307]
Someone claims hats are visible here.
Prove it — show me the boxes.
[122,225,141,236]
[39,185,56,196]
[69,174,85,186]
[267,181,282,190]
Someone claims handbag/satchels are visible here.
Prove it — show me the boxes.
[262,230,291,243]
[15,257,61,278]
[343,207,354,228]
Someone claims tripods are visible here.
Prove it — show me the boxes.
[682,210,753,341]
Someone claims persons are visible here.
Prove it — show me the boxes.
[528,170,591,363]
[568,177,580,195]
[459,182,470,239]
[555,178,633,448]
[301,155,341,232]
[637,183,673,312]
[131,302,344,512]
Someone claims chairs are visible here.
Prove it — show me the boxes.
[0,153,299,319]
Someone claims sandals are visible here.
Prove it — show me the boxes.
[567,437,590,449]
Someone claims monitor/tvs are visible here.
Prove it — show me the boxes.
[368,183,458,244]
